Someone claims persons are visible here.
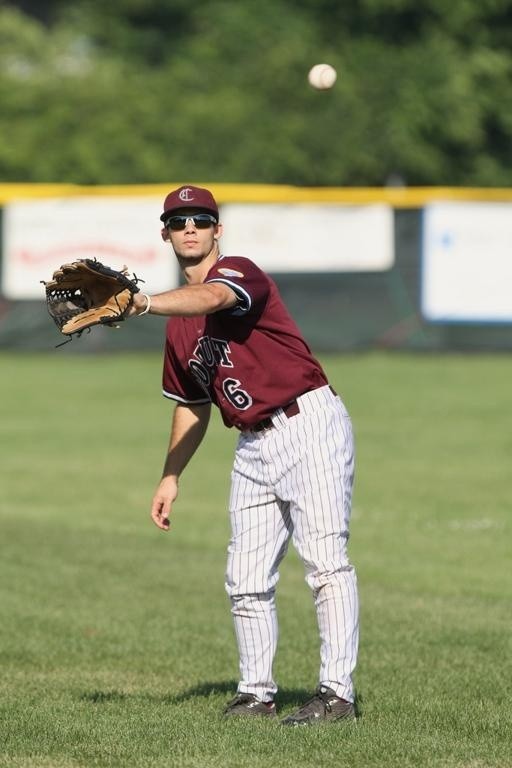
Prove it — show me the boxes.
[43,185,361,725]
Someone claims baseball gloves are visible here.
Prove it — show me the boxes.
[41,258,145,348]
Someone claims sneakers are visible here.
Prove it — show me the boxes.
[282,685,357,728]
[222,693,277,720]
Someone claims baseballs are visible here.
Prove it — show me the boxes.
[308,64,337,89]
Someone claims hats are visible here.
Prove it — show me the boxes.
[160,186,218,223]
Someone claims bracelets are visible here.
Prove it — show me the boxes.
[134,291,153,321]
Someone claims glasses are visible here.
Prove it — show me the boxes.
[164,213,217,229]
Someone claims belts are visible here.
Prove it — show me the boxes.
[250,386,338,433]
